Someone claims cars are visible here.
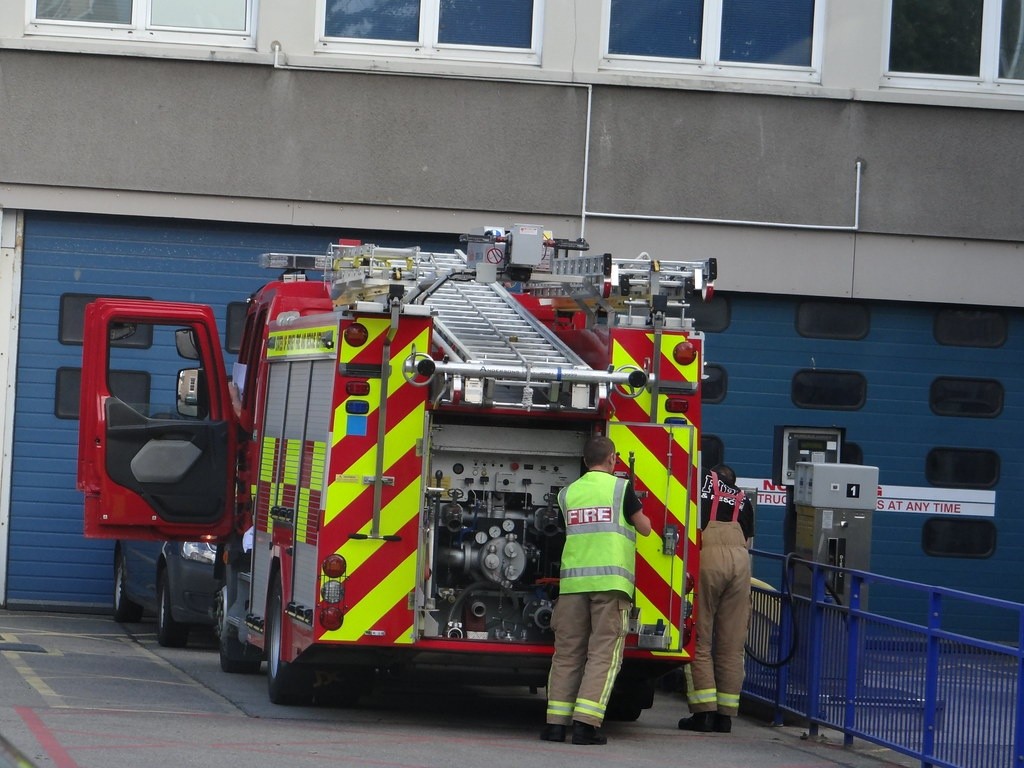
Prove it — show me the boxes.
[113,540,226,648]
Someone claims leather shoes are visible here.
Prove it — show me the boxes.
[571,719,608,746]
[679,711,712,732]
[712,715,731,732]
[540,723,567,742]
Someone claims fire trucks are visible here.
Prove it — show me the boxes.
[76,223,718,709]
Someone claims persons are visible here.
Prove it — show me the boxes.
[540,436,651,745]
[676,466,754,733]
